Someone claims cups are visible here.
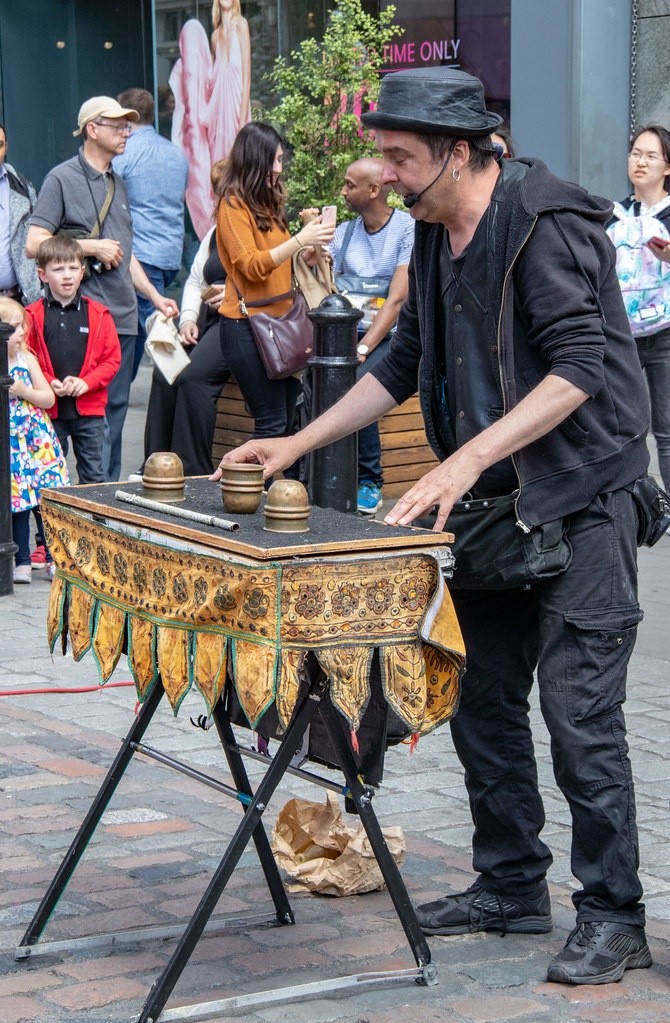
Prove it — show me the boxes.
[262,479,311,534]
[142,452,188,503]
[218,463,266,514]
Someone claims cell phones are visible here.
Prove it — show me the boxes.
[647,236,670,250]
[201,286,222,301]
[322,206,337,227]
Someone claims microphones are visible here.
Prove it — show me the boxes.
[403,151,451,208]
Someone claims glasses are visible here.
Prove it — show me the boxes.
[97,122,132,133]
[626,151,663,162]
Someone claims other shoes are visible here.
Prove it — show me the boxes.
[48,562,58,581]
[14,565,32,584]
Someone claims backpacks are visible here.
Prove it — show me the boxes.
[605,196,670,337]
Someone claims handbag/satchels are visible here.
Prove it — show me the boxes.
[82,258,92,282]
[635,476,670,548]
[408,488,571,592]
[241,286,314,379]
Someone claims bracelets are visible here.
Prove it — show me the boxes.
[295,235,302,247]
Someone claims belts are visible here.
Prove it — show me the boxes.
[0,288,20,299]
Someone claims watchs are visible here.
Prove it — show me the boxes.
[356,345,371,356]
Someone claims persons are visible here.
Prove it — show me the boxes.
[168,0,252,244]
[325,9,370,70]
[490,131,514,158]
[129,120,416,515]
[0,88,188,585]
[602,125,670,538]
[157,85,175,118]
[205,68,652,984]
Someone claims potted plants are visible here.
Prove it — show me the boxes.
[211,0,441,500]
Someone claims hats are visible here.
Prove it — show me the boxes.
[72,96,140,138]
[361,66,504,138]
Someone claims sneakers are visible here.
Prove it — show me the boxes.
[547,922,653,985]
[30,545,47,569]
[356,479,384,514]
[414,875,553,936]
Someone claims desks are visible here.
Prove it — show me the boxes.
[14,480,468,1022]
